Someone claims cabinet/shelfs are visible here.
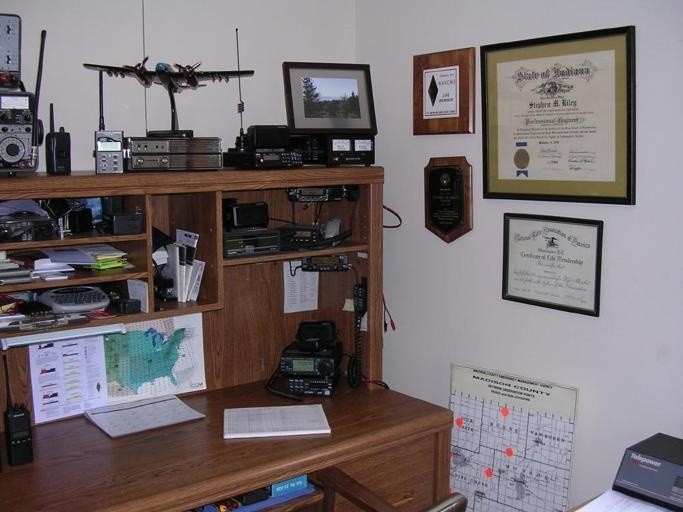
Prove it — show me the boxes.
[0,171,384,341]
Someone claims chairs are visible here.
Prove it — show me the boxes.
[310,464,469,512]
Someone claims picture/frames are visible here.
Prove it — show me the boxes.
[410,46,478,139]
[477,25,636,205]
[501,213,604,317]
[280,58,381,138]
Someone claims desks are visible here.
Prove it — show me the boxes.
[2,376,452,512]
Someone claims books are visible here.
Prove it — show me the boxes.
[0,241,130,286]
[163,227,205,302]
[222,401,331,439]
